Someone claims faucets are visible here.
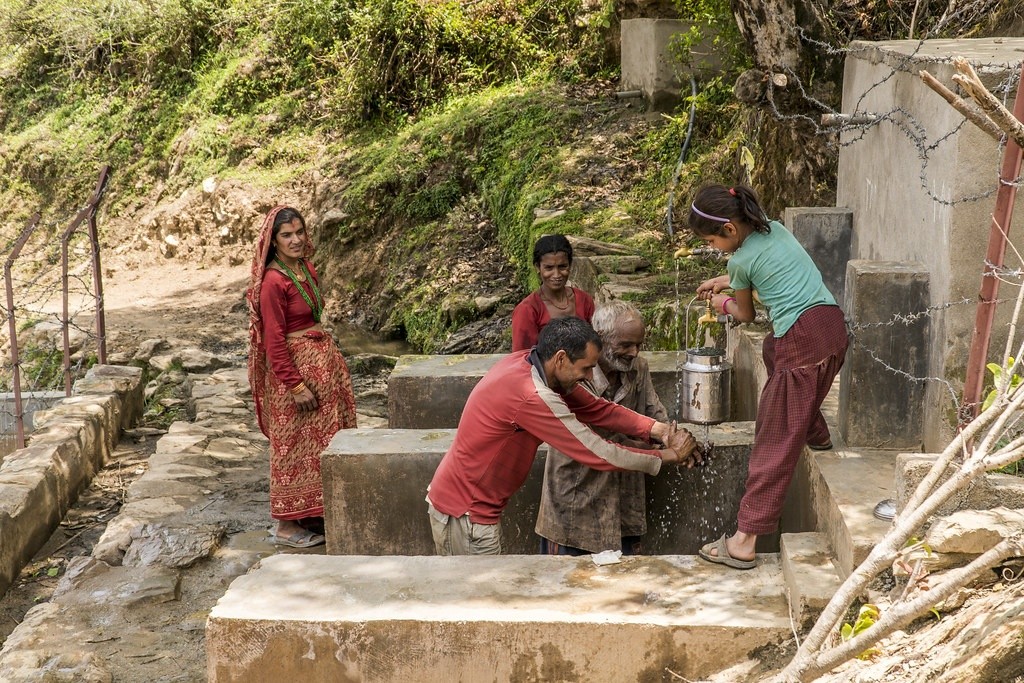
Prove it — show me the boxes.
[696,299,718,326]
[673,234,693,260]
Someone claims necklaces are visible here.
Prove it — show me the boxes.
[275,254,302,280]
[541,285,570,310]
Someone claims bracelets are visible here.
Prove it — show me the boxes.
[722,298,737,314]
[291,382,306,395]
[672,449,680,463]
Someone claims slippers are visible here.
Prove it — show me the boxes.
[276,529,325,547]
[699,532,756,569]
[808,437,833,450]
[299,520,325,533]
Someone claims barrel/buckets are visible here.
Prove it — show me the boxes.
[682,294,733,425]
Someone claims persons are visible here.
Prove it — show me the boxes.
[688,184,850,570]
[534,300,679,555]
[248,206,357,548]
[512,234,596,352]
[425,317,704,556]
